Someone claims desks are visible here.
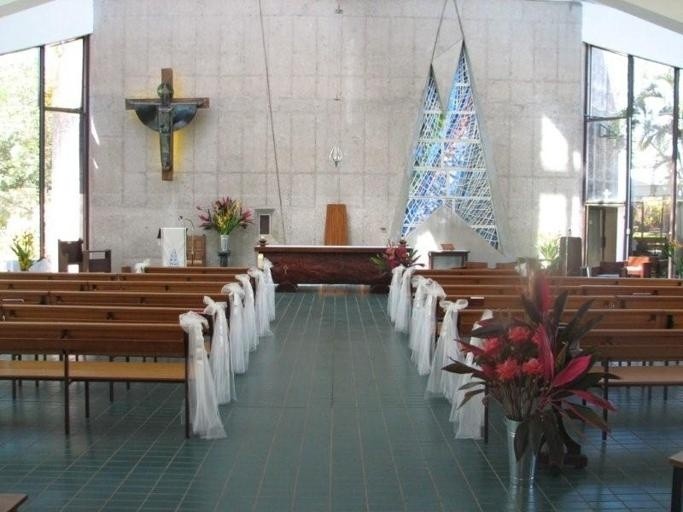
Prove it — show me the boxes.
[429,252,468,270]
[254,244,412,294]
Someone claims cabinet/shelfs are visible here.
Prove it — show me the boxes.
[83,250,112,273]
[187,233,207,265]
[58,239,84,273]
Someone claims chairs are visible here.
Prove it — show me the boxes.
[621,255,652,279]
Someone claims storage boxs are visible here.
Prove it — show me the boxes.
[668,451,683,512]
[0,493,27,511]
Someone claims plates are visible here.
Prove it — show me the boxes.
[83,250,112,273]
[187,233,207,265]
[58,239,84,273]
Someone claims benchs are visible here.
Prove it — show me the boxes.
[668,451,683,512]
[390,266,682,439]
[0,493,27,511]
[0,265,254,432]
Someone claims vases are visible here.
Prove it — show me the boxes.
[500,416,545,486]
[196,195,257,233]
[369,237,424,279]
[9,232,45,270]
[440,264,619,479]
[219,234,231,255]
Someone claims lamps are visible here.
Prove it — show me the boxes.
[621,255,652,279]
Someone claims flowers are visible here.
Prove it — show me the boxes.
[369,237,424,279]
[196,195,257,233]
[440,264,619,479]
[9,232,45,270]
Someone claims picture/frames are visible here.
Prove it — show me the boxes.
[254,244,412,294]
[429,252,468,270]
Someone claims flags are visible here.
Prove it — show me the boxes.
[500,416,545,486]
[219,234,231,255]
